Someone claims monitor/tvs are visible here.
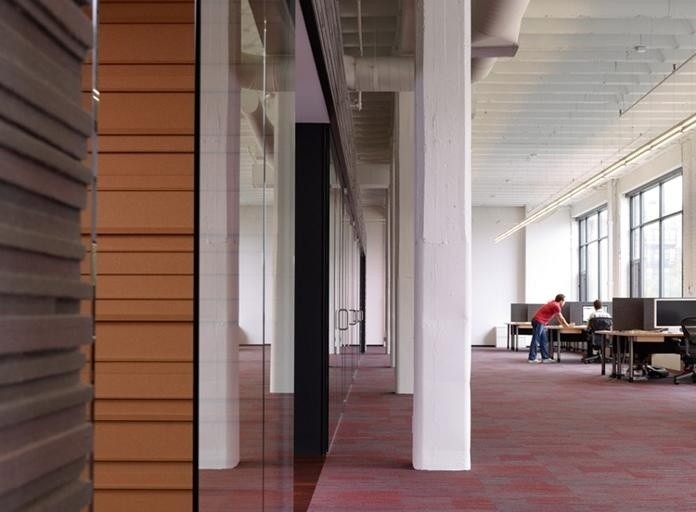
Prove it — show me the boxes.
[582,305,608,323]
[653,299,696,334]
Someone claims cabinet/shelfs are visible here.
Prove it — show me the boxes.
[295,122,362,512]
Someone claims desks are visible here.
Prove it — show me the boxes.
[546,324,587,362]
[595,328,688,382]
[505,322,534,352]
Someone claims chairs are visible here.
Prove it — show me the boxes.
[583,318,614,364]
[674,316,696,385]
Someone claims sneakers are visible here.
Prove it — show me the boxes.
[528,359,541,363]
[543,358,555,363]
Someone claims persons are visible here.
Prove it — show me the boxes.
[528,294,576,365]
[586,299,613,356]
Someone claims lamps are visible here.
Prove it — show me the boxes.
[493,58,696,240]
